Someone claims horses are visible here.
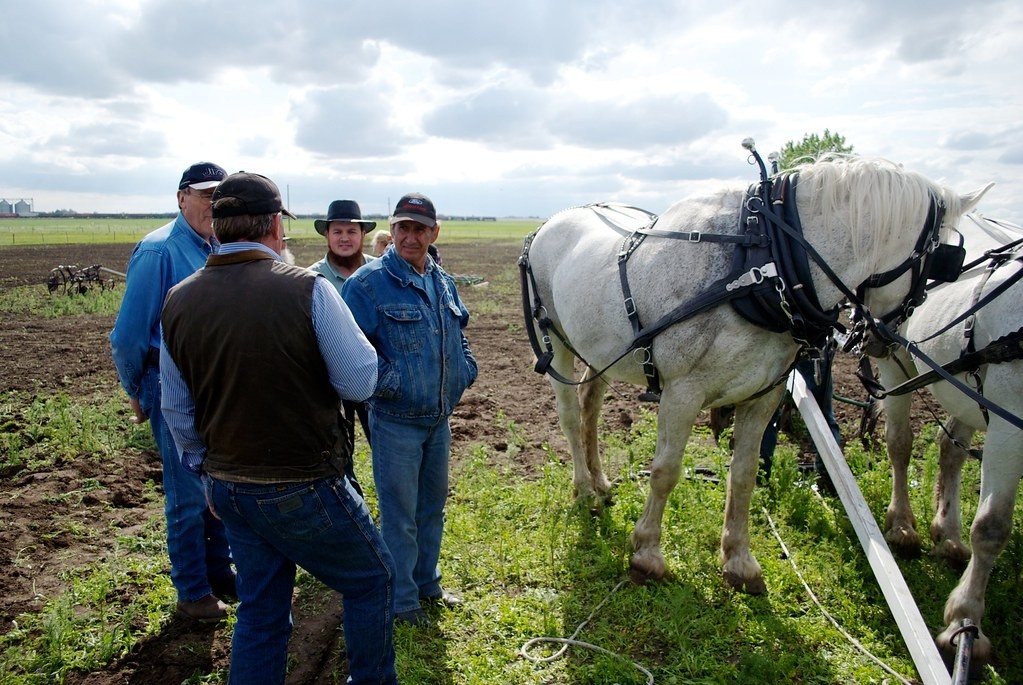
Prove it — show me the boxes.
[516,149,996,599]
[873,210,1023,657]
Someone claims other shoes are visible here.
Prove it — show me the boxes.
[425,591,460,607]
[176,594,231,623]
[213,572,237,596]
[400,609,429,627]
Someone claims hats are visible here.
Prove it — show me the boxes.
[179,162,228,190]
[390,192,436,227]
[211,171,297,220]
[313,200,376,237]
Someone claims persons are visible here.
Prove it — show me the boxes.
[159,170,397,685]
[303,199,382,500]
[108,162,243,619]
[339,193,478,630]
